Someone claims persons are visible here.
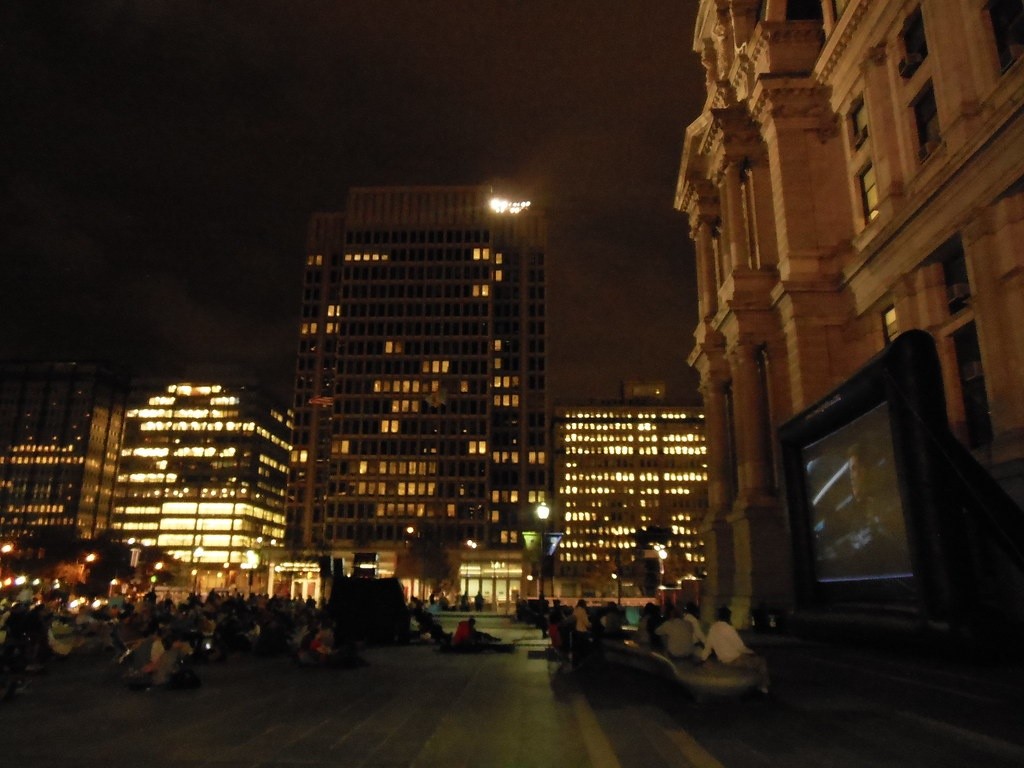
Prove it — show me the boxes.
[452,618,502,646]
[536,600,625,649]
[639,601,706,660]
[430,591,484,612]
[1,585,340,693]
[699,606,772,694]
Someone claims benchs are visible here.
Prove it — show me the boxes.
[568,630,763,701]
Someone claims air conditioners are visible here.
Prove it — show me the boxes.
[1000,44,1024,71]
[917,140,937,164]
[897,52,923,76]
[945,283,966,304]
[963,362,983,382]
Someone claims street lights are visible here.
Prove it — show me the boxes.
[536,501,551,636]
